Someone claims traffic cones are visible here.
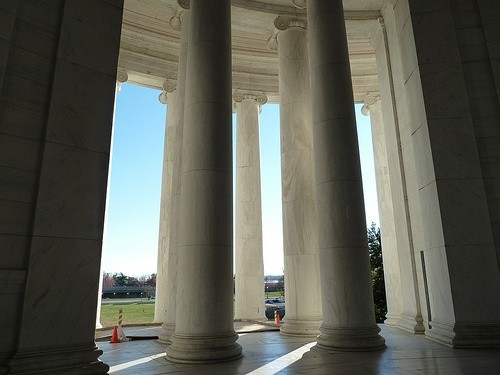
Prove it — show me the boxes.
[109,325,121,343]
[275,314,281,324]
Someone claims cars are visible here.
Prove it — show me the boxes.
[266,297,285,303]
